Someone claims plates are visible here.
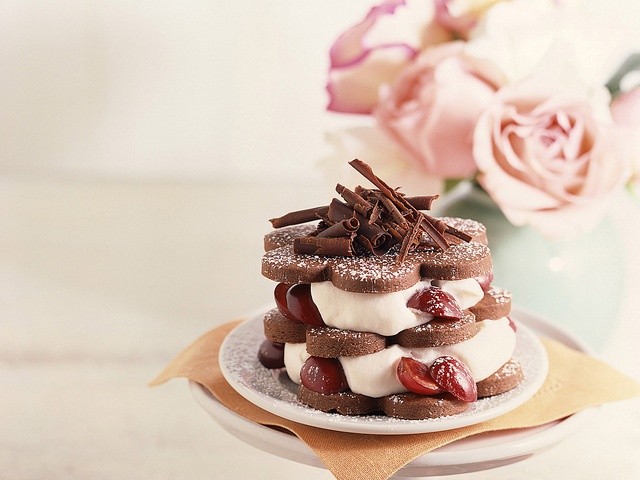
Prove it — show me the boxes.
[193,313,593,467]
[219,314,550,434]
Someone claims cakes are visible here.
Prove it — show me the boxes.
[257,159,524,419]
